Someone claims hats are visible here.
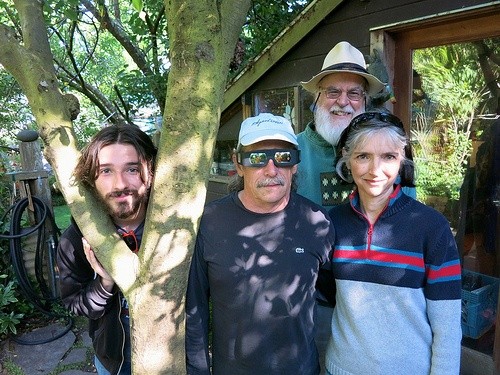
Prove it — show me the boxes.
[237,113,299,152]
[299,41,387,97]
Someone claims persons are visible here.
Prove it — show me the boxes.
[324,112,464,375]
[293,41,414,216]
[184,113,336,375]
[56,125,158,375]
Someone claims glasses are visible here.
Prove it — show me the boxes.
[237,150,301,167]
[344,112,405,133]
[318,87,366,101]
